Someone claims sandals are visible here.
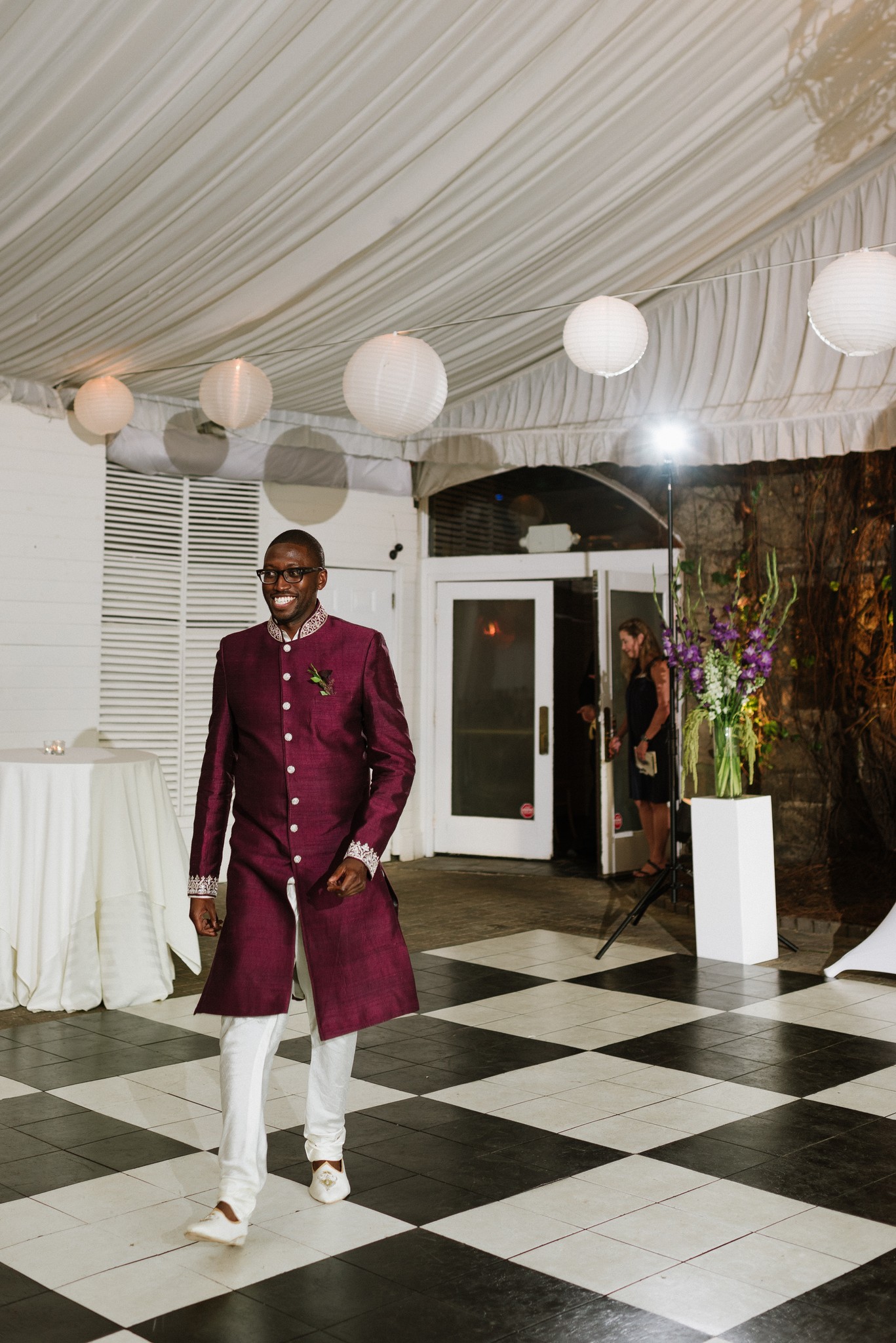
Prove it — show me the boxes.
[632,858,670,878]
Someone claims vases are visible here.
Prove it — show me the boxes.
[712,724,743,798]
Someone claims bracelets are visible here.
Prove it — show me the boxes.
[642,735,650,743]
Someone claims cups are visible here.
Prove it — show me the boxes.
[44,739,66,755]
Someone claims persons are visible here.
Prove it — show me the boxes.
[607,620,671,878]
[578,704,595,723]
[183,530,419,1249]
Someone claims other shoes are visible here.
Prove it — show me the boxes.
[309,1159,351,1205]
[183,1208,248,1247]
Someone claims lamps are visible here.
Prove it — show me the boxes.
[197,358,273,430]
[807,249,896,359]
[563,296,649,378]
[73,376,136,434]
[341,333,447,439]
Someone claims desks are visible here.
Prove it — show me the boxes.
[1,745,204,1014]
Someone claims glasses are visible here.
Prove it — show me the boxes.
[256,567,323,584]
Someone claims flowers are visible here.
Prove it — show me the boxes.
[651,546,801,800]
